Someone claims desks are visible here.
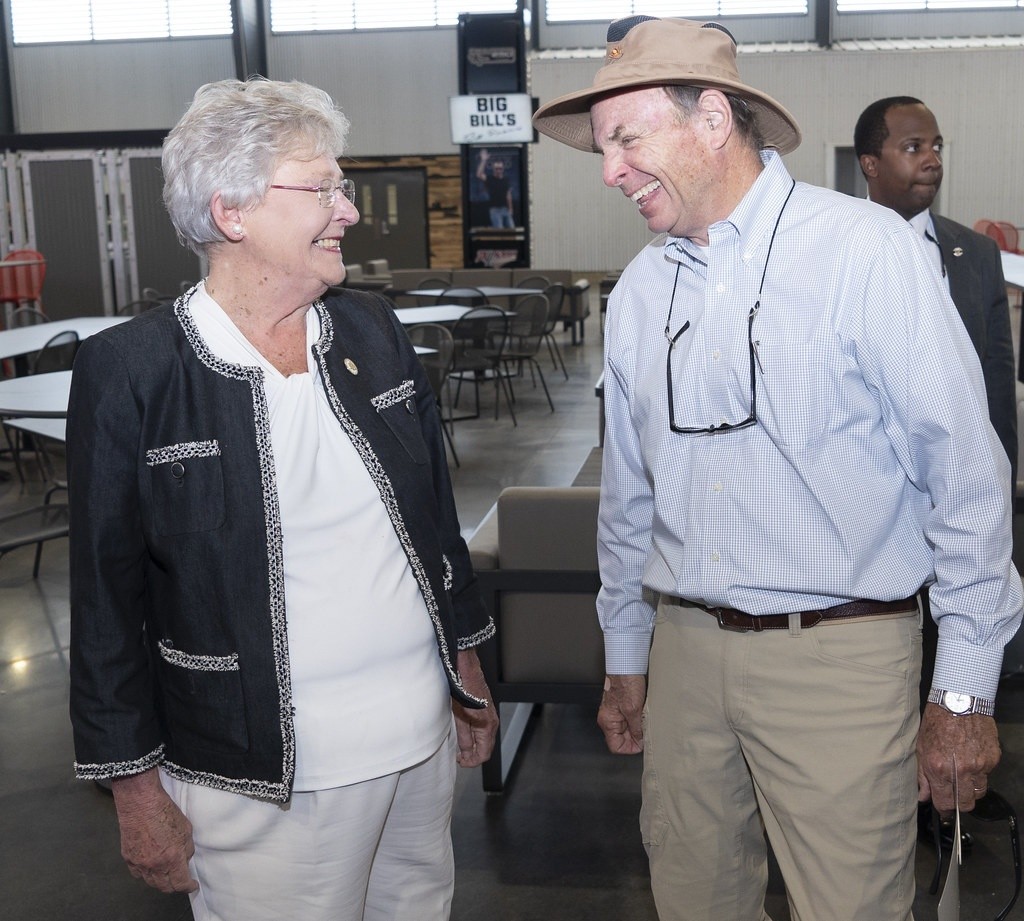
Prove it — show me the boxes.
[0,370,73,417]
[392,305,517,423]
[0,315,138,464]
[405,287,544,382]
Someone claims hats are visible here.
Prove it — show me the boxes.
[531,14,802,156]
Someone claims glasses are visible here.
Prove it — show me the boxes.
[666,300,759,438]
[261,178,354,209]
[929,788,1021,921]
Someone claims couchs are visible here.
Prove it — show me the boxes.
[464,445,606,794]
[366,259,591,346]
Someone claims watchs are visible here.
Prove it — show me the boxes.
[926,688,995,716]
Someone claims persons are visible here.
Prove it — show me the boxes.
[531,18,1024,921]
[853,96,1018,862]
[476,148,514,229]
[62,76,502,921]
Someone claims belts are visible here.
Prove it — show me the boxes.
[681,593,918,633]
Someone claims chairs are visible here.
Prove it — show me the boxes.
[974,219,1024,308]
[0,273,568,577]
